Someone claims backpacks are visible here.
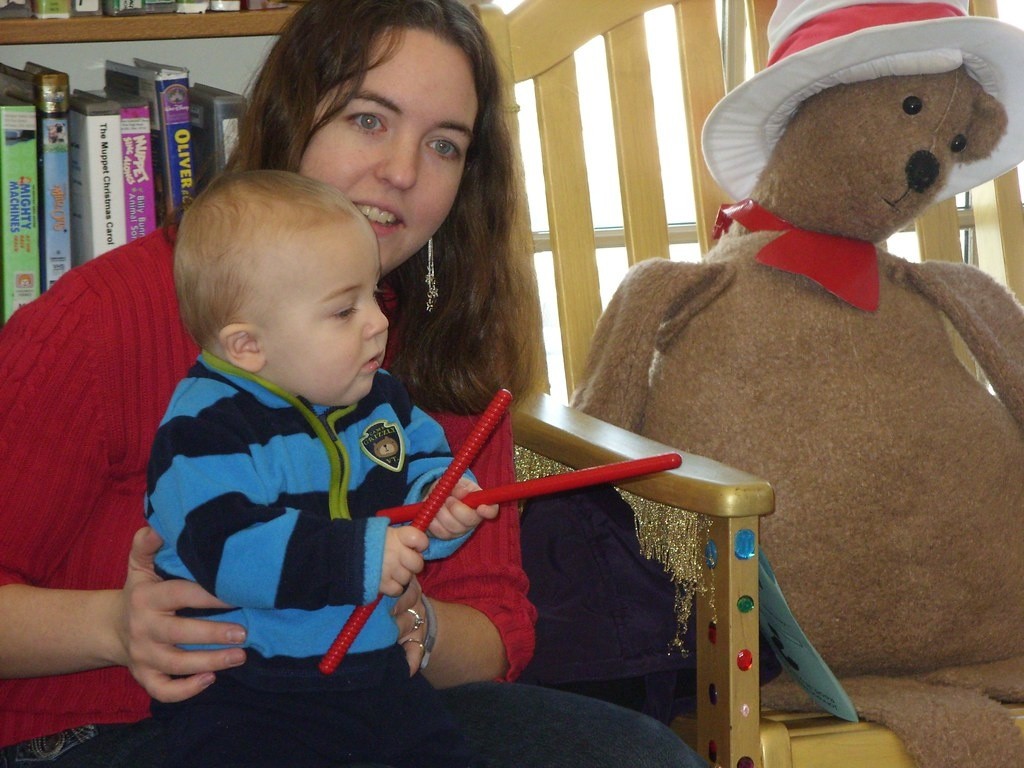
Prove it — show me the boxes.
[520,490,681,718]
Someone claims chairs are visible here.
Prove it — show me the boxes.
[470,1,1023,768]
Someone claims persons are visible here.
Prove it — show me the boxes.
[144,172,502,768]
[0,0,709,768]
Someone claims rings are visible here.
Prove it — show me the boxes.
[408,608,425,633]
[407,638,425,655]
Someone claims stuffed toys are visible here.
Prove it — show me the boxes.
[568,0,1024,768]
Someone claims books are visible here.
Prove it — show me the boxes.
[0,0,289,322]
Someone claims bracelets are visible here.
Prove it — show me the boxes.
[420,591,438,670]
